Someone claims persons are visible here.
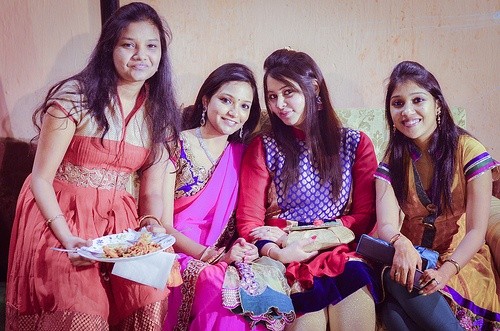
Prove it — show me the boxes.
[162,62,296,331]
[373,60,500,331]
[236,45,379,331]
[5,2,180,331]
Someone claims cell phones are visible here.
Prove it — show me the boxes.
[406,269,424,291]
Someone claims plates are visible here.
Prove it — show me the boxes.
[77,232,176,262]
[283,224,331,230]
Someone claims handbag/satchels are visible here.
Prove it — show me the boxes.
[355,234,439,274]
[279,223,355,254]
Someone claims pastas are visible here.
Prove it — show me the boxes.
[103,240,160,258]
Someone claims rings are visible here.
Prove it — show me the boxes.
[395,272,400,275]
[435,279,440,284]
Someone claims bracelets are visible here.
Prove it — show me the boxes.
[200,245,210,261]
[390,233,401,244]
[444,258,460,274]
[139,214,161,225]
[46,213,64,225]
[267,244,277,258]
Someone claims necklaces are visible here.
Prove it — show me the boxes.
[196,127,226,171]
